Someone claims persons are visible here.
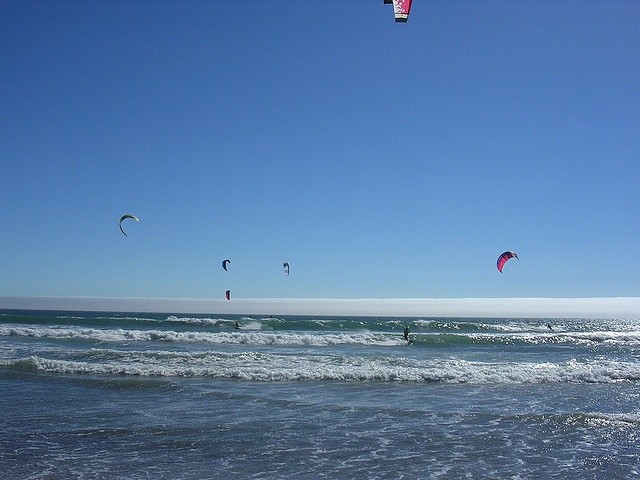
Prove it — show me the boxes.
[403,326,411,341]
[546,322,554,331]
[234,321,239,328]
